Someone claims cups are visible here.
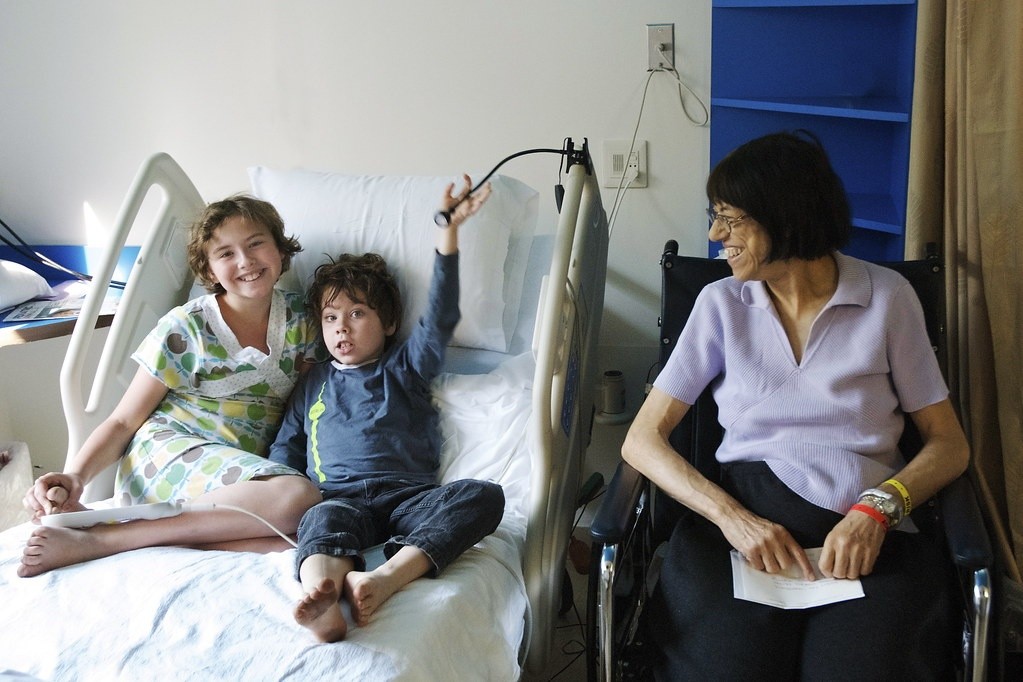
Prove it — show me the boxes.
[602,370,625,414]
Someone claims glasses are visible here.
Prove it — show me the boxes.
[707,208,763,232]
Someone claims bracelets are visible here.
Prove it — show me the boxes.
[884,479,912,517]
[858,488,904,527]
[850,503,890,534]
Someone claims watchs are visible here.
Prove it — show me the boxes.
[862,496,899,526]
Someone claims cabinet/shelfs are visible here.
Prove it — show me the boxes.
[708,0,934,266]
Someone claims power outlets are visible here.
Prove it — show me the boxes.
[647,23,674,70]
[603,141,650,189]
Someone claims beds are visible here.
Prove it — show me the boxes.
[0,141,609,682]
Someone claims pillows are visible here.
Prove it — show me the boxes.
[235,160,532,354]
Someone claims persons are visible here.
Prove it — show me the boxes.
[268,173,506,644]
[621,129,973,682]
[17,196,325,580]
